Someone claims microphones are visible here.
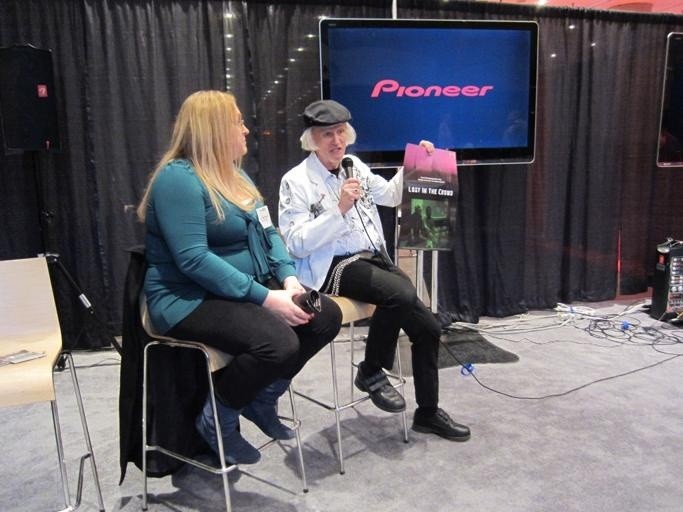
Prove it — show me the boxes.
[342,156,354,178]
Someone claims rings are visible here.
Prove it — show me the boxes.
[354,188,358,194]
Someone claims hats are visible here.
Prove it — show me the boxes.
[303,99,352,128]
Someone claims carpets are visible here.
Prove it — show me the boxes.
[391,328,518,378]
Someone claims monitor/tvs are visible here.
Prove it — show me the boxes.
[656,30,683,169]
[319,16,540,169]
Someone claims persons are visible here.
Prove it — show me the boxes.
[411,204,430,242]
[422,205,440,249]
[143,90,342,465]
[279,99,471,442]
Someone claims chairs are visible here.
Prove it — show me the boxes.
[0,257,105,511]
[133,246,309,511]
[293,295,408,474]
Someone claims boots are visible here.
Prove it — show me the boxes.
[240,373,296,442]
[195,395,262,466]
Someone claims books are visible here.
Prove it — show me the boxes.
[0,349,45,364]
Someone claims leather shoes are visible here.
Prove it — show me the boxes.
[411,405,471,442]
[354,361,408,413]
